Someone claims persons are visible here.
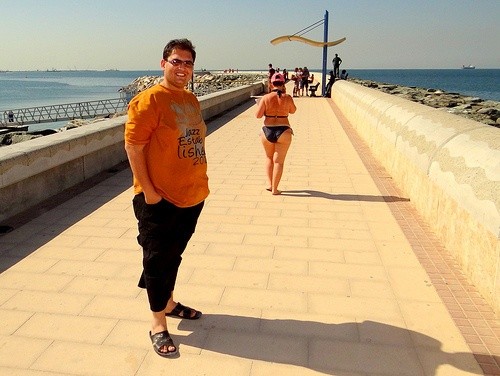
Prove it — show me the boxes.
[340,70,348,80]
[292,68,300,97]
[323,70,336,98]
[123,37,210,357]
[268,64,275,93]
[223,68,239,73]
[255,71,297,196]
[8,109,15,123]
[275,67,314,83]
[301,67,309,97]
[332,53,342,79]
[298,67,303,97]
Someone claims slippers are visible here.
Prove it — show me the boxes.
[150,331,178,357]
[164,302,202,320]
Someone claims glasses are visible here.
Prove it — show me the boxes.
[166,58,194,69]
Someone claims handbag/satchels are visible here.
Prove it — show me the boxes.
[291,75,298,82]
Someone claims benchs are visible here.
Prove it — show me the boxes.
[309,82,320,97]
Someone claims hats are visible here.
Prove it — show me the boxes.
[271,73,284,83]
